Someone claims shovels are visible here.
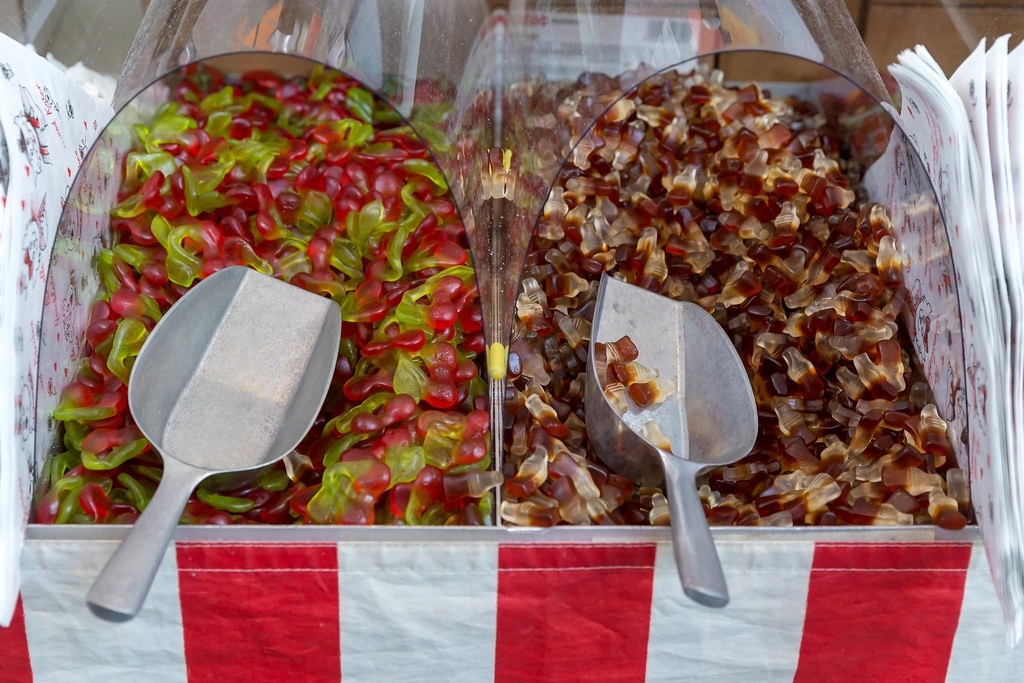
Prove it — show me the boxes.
[87,263,343,625]
[587,271,758,609]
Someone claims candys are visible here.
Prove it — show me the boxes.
[34,56,979,533]
